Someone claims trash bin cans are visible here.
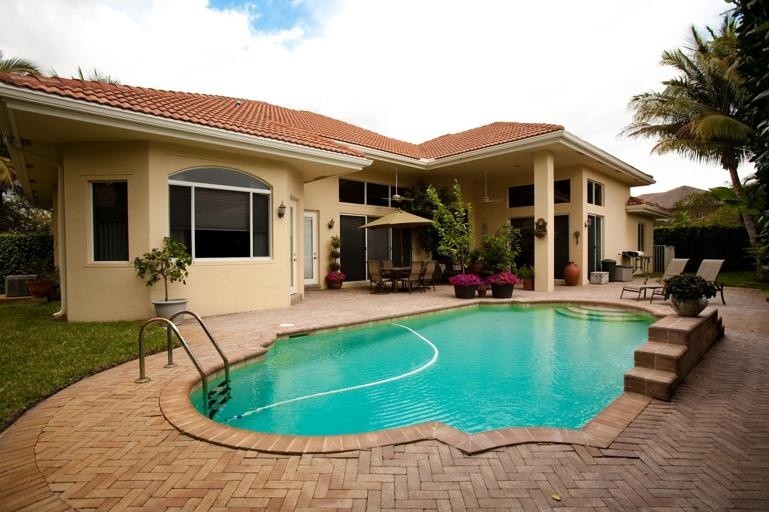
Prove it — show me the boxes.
[601,258,617,282]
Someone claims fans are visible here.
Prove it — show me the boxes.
[381,167,415,203]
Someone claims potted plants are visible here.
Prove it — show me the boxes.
[663,274,716,317]
[129,237,193,327]
[26,260,59,298]
[518,263,535,290]
[471,250,484,272]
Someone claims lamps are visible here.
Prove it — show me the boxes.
[277,200,287,218]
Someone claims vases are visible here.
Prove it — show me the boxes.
[328,281,342,289]
[454,284,476,298]
[478,289,487,296]
[491,283,514,298]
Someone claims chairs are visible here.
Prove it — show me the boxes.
[367,258,437,295]
[620,258,727,305]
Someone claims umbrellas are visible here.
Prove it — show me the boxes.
[359,211,434,266]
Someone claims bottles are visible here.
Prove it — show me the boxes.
[563,261,581,286]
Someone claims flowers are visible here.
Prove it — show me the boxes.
[447,272,519,289]
[325,272,346,281]
